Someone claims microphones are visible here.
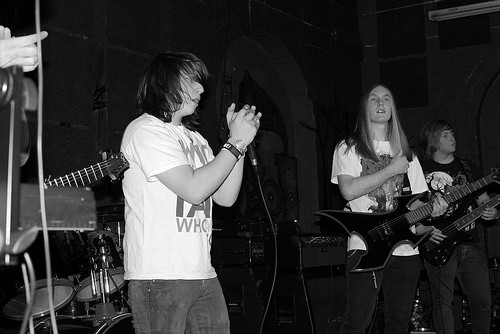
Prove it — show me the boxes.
[247,140,257,166]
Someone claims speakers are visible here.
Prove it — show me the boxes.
[217,263,266,334]
[299,277,350,334]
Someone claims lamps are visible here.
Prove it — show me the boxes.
[428,0,500,22]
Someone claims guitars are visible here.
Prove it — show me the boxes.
[6,151,130,254]
[417,194,500,267]
[314,167,500,272]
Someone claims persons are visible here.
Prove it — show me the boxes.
[411,118,500,334]
[0,25,75,168]
[119,51,262,334]
[330,84,449,334]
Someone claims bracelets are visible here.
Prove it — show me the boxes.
[221,143,242,160]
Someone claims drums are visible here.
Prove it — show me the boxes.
[23,313,136,334]
[1,232,75,320]
[66,231,127,302]
